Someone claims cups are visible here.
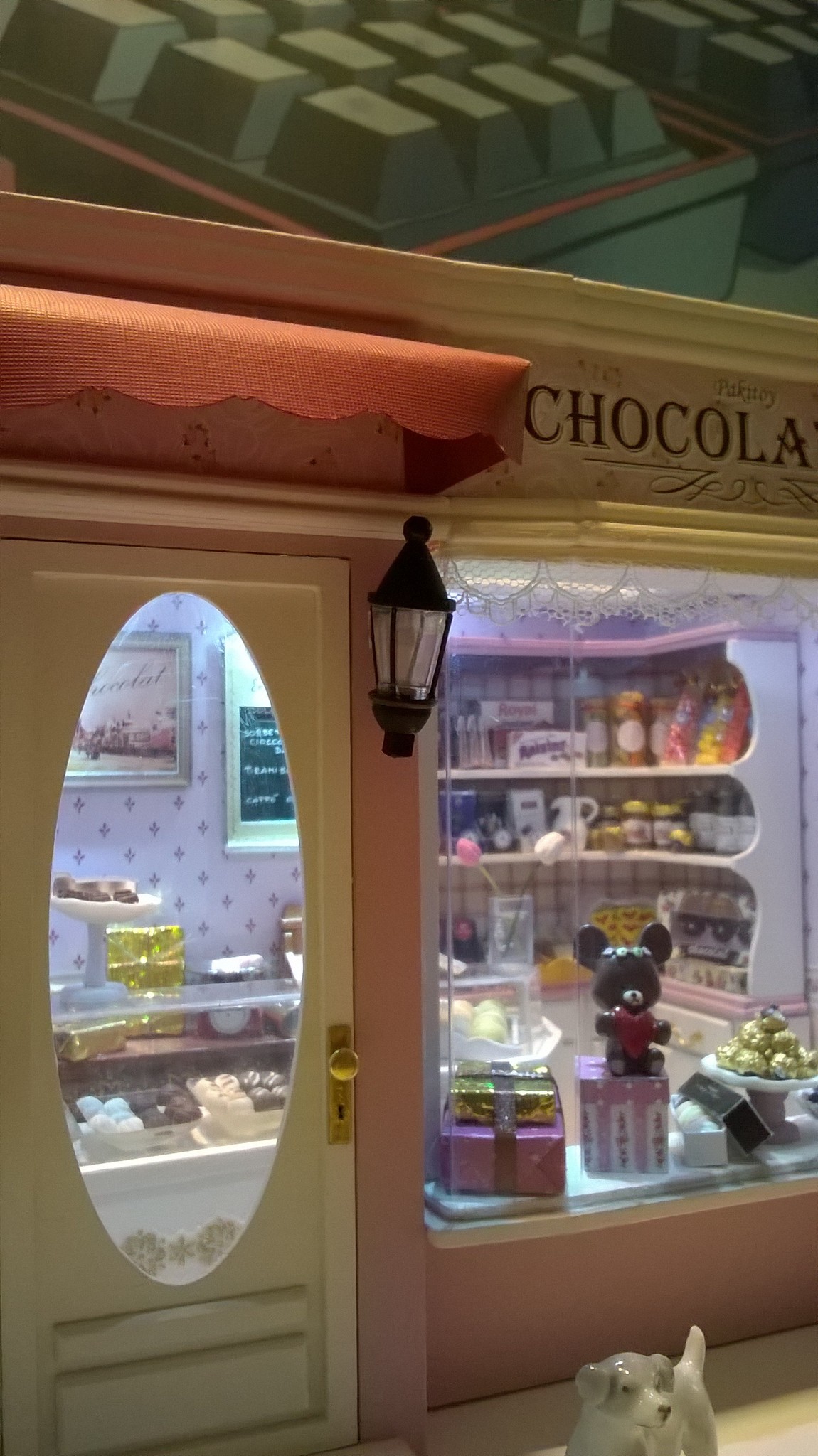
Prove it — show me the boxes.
[485,894,533,973]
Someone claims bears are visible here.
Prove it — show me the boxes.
[575,922,672,1076]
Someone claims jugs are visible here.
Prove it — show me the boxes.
[548,794,600,852]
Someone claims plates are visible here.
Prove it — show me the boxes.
[75,1090,203,1152]
[185,1072,289,1135]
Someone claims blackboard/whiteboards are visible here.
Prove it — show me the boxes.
[237,705,298,822]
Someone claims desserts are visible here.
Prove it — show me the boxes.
[437,997,510,1043]
[56,888,138,905]
[71,1067,289,1134]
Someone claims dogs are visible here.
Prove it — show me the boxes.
[563,1326,717,1456]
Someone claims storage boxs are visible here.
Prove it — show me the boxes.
[670,1072,774,1166]
[576,1056,671,1172]
[51,1022,127,1061]
[107,924,184,1036]
[442,1062,565,1195]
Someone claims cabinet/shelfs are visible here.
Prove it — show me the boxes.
[439,620,816,1060]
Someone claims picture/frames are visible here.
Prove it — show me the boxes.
[63,632,194,784]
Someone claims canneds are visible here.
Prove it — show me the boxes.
[581,801,698,851]
[580,690,675,769]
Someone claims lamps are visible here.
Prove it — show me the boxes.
[362,517,456,756]
